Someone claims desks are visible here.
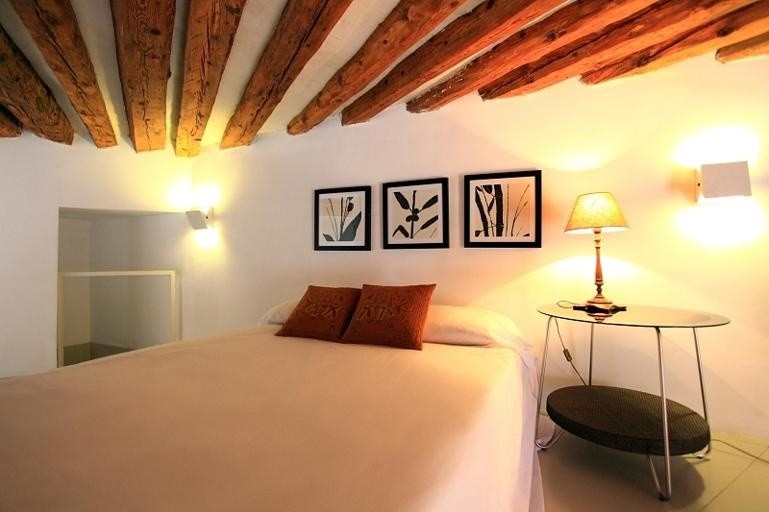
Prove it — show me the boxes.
[536,306,731,501]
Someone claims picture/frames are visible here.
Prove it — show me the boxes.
[314,186,371,251]
[382,177,449,250]
[464,169,541,248]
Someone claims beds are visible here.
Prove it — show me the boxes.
[0,323,544,512]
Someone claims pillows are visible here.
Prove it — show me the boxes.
[257,298,519,347]
[339,284,437,350]
[274,285,362,344]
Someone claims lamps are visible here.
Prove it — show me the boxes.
[692,160,751,203]
[564,192,628,314]
